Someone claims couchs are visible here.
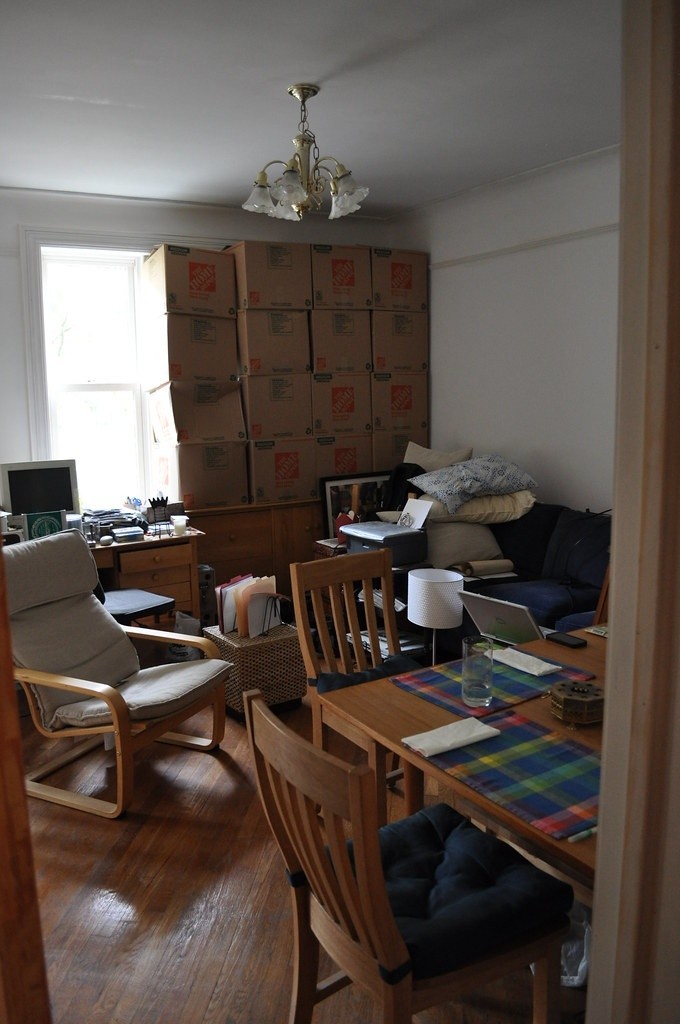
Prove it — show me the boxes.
[346,503,614,632]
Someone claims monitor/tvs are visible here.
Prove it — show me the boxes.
[0,459,81,529]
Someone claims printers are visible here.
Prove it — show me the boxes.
[339,513,430,566]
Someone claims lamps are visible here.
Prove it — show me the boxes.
[406,566,464,668]
[241,85,370,222]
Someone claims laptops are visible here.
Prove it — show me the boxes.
[456,590,558,645]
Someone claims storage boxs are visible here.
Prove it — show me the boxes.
[141,236,432,513]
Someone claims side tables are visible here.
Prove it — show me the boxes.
[312,540,348,619]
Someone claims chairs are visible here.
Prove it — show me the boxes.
[92,578,177,626]
[0,527,236,820]
[240,688,572,1024]
[289,547,402,828]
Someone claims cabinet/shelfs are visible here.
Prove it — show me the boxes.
[86,521,206,655]
[184,500,330,628]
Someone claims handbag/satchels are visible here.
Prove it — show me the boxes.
[529,920,592,987]
[162,610,201,662]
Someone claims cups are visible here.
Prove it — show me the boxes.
[173,519,186,536]
[82,521,101,543]
[461,635,493,708]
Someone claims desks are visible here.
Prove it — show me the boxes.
[316,620,608,910]
[202,620,308,712]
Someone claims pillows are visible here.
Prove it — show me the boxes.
[378,441,540,570]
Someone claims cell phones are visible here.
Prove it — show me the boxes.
[546,632,587,649]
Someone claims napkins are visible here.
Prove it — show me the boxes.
[484,646,565,677]
[400,716,502,758]
